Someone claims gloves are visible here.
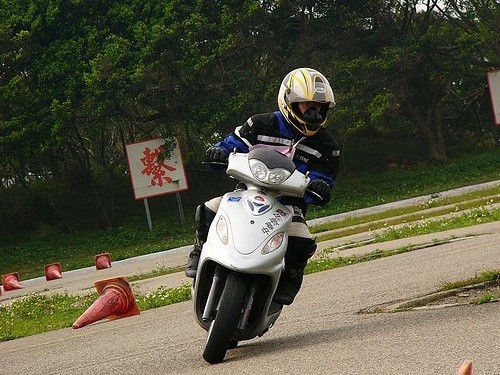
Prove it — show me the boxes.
[206,145,227,163]
[306,179,330,206]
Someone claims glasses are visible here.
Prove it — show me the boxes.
[299,102,323,109]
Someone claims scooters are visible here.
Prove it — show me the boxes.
[191,147,317,364]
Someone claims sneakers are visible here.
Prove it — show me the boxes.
[276,294,292,305]
[186,249,201,277]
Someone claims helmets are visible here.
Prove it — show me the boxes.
[278,67,336,136]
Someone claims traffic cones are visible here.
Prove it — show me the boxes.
[72,276,141,329]
[93,252,112,271]
[45,262,63,281]
[1,272,23,292]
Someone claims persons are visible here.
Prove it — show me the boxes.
[185,67,342,305]
